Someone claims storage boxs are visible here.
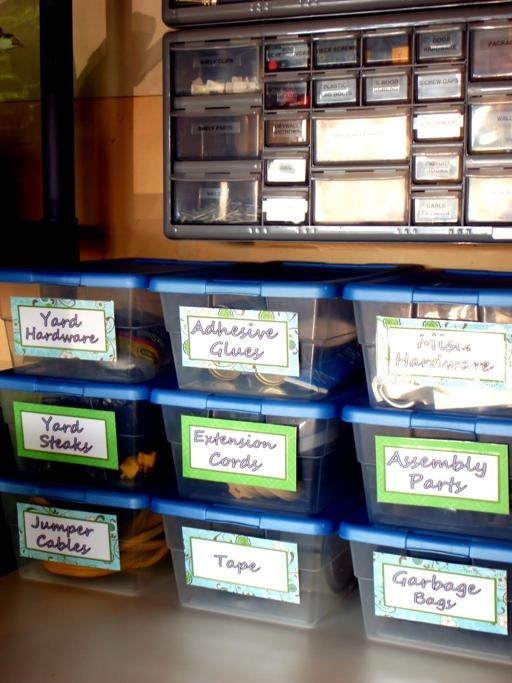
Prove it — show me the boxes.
[338,267,511,665]
[0,258,154,598]
[150,263,395,627]
